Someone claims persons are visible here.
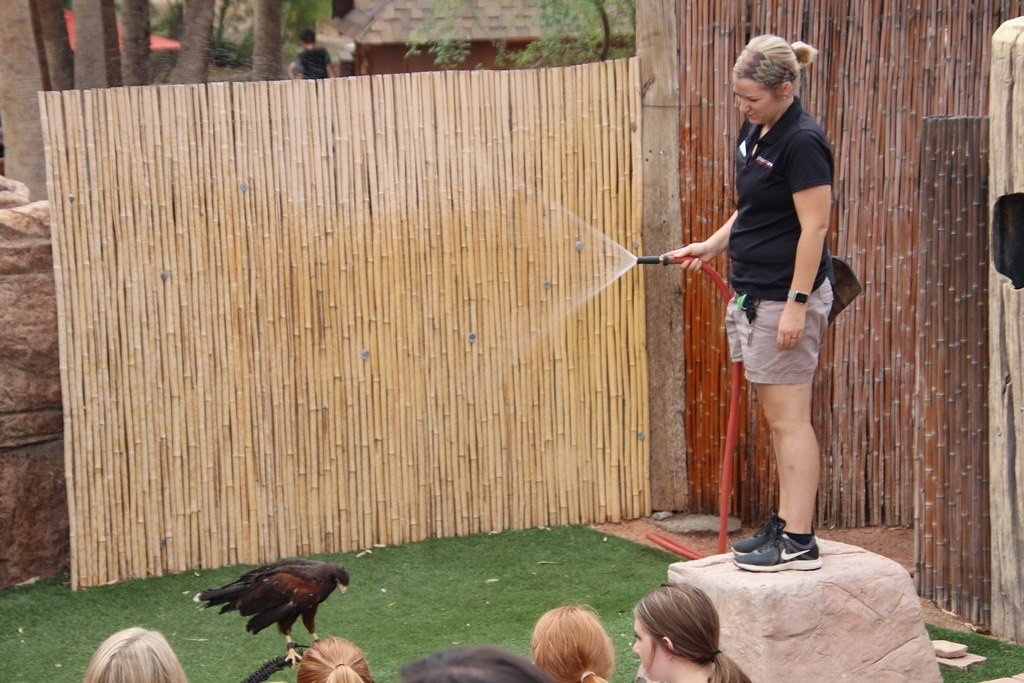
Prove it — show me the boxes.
[288,29,336,80]
[529,606,615,683]
[658,35,833,574]
[399,644,556,683]
[632,582,753,683]
[297,637,372,683]
[82,628,188,683]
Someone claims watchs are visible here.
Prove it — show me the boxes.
[788,290,810,304]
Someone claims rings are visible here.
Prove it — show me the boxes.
[791,336,797,339]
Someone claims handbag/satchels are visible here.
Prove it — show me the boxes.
[828,254,863,327]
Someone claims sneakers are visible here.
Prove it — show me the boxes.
[730,515,779,556]
[733,529,823,572]
